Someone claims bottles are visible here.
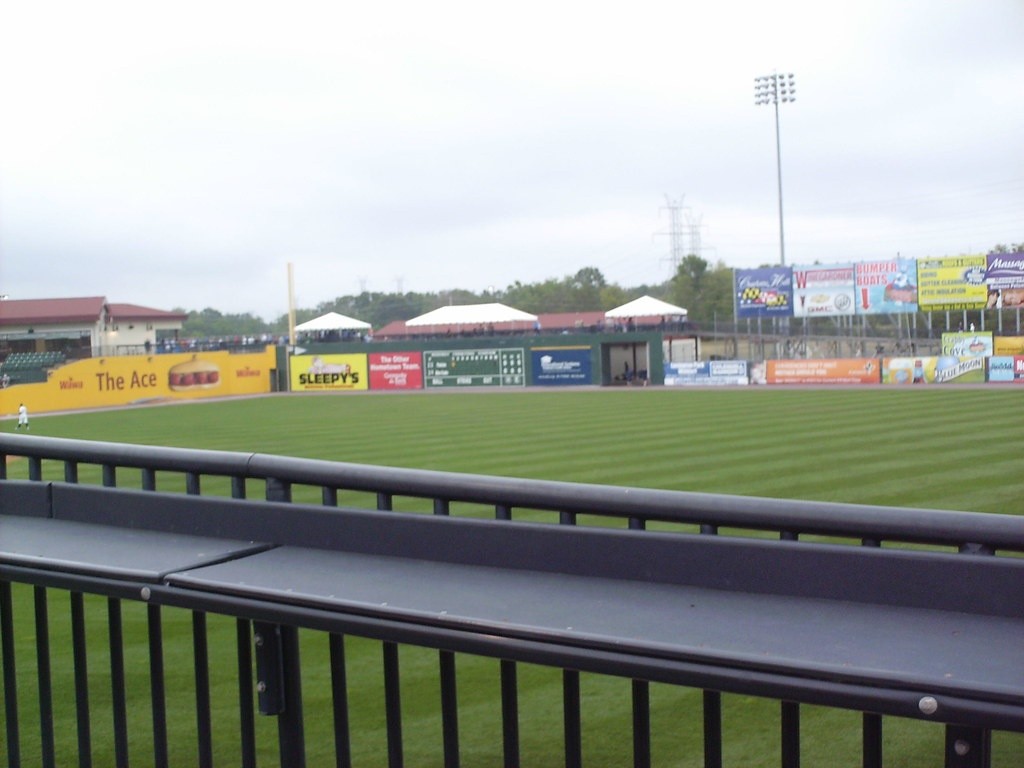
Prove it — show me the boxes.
[913,360,927,384]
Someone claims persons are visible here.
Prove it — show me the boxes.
[144,315,683,353]
[987,289,1000,309]
[958,321,963,333]
[876,343,884,354]
[970,322,975,332]
[624,361,634,387]
[15,404,29,430]
[0,373,10,389]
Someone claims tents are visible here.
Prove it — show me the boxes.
[604,295,688,328]
[405,302,538,339]
[294,312,371,342]
